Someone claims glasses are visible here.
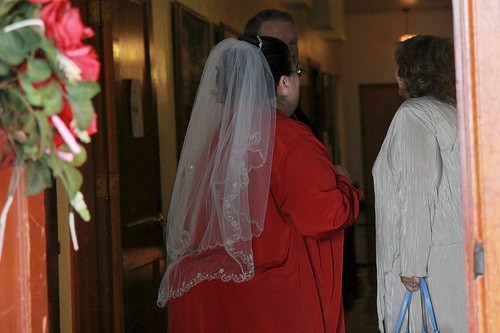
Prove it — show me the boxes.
[290,66,304,76]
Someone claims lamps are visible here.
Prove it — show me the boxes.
[396,1,416,42]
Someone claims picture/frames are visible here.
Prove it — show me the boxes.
[218,21,242,42]
[172,1,212,164]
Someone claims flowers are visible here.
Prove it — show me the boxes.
[12,1,103,147]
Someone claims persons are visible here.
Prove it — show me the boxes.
[155,31,365,333]
[243,8,329,146]
[372,32,471,333]
